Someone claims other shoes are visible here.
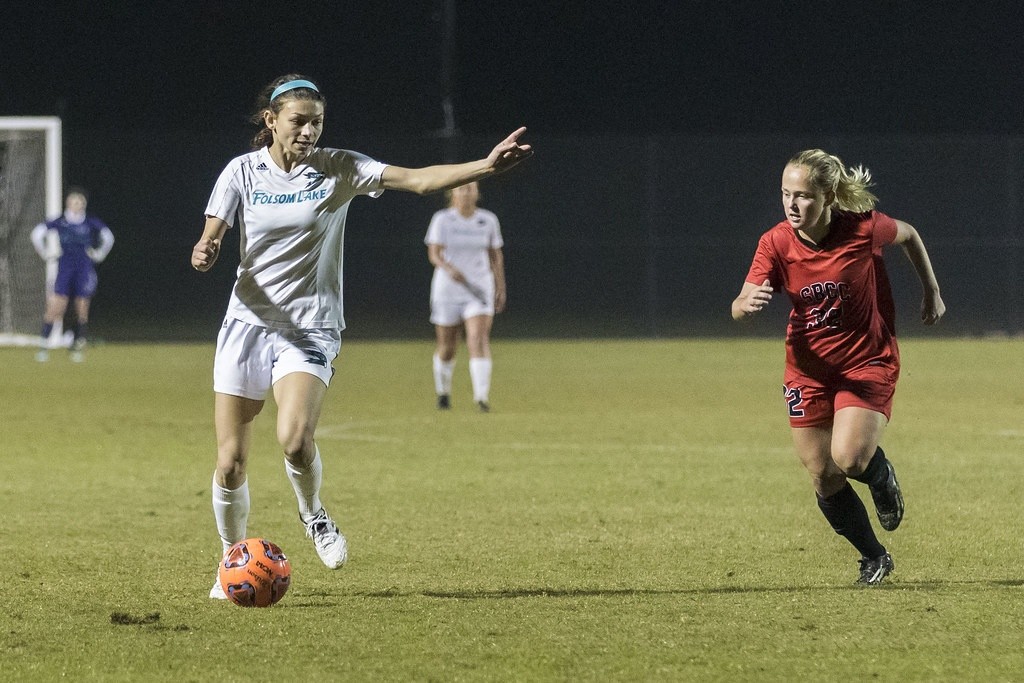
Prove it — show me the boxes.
[437,395,450,410]
[477,399,491,411]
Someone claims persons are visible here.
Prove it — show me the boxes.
[730,148,948,586]
[424,183,507,412]
[27,192,116,361]
[192,72,534,602]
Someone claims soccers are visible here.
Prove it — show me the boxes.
[218,538,290,608]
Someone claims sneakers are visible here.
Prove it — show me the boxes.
[299,506,348,570]
[209,562,228,599]
[853,545,894,586]
[868,458,905,532]
